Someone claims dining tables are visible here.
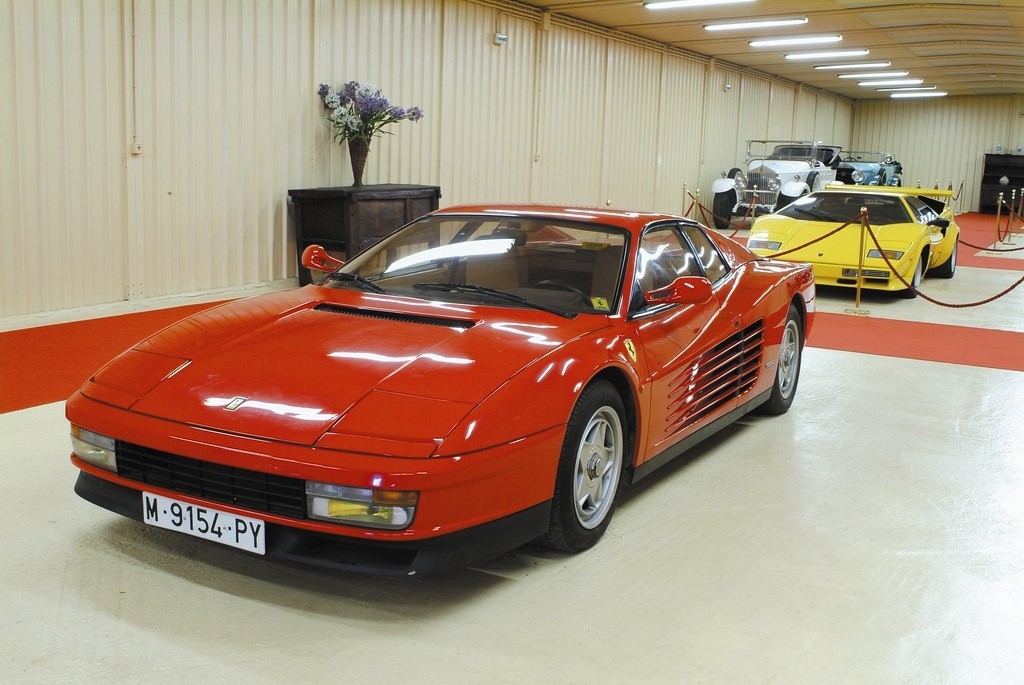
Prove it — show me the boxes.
[880,203,900,222]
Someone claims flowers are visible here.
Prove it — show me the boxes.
[317,80,425,151]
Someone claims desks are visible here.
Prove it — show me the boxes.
[288,183,443,287]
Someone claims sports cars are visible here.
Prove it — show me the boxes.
[65,203,815,576]
[745,178,961,299]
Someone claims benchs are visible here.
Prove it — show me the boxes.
[777,148,834,164]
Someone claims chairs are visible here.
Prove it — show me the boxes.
[591,245,655,303]
[465,235,532,290]
[846,200,866,209]
[819,199,845,211]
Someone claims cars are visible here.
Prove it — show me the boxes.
[712,140,904,230]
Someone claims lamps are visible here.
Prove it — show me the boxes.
[642,0,757,12]
[748,33,842,47]
[784,49,869,60]
[857,78,924,86]
[813,60,892,70]
[874,85,937,92]
[702,15,808,31]
[837,71,909,79]
